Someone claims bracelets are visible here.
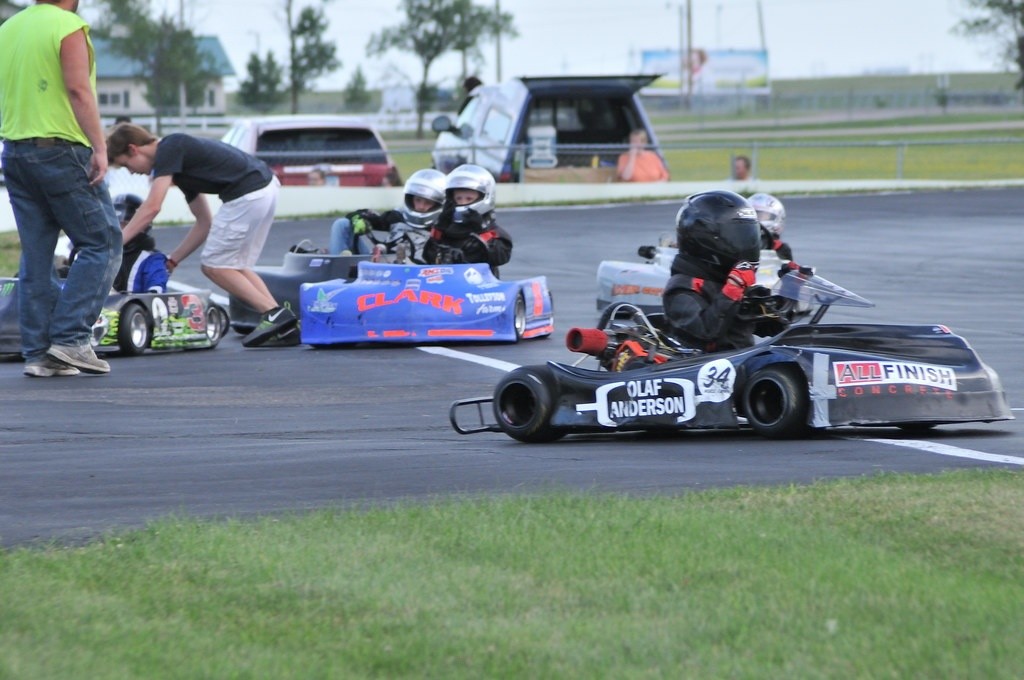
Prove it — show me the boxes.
[166,254,177,267]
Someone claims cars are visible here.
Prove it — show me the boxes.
[428,71,673,184]
[219,112,404,188]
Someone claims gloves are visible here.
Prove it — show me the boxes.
[438,202,453,227]
[463,209,482,231]
[777,262,813,295]
[722,259,757,302]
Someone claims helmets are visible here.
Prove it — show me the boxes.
[446,163,498,223]
[111,195,152,237]
[746,193,785,235]
[676,191,761,276]
[401,168,448,228]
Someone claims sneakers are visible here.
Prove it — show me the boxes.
[262,328,301,347]
[47,343,111,374]
[241,301,298,348]
[24,357,80,378]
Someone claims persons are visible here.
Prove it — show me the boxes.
[617,130,670,182]
[112,193,168,294]
[381,172,397,187]
[742,193,794,260]
[0,0,127,377]
[421,164,513,281]
[106,124,301,347]
[114,116,133,131]
[330,168,446,263]
[661,188,803,356]
[308,169,325,185]
[722,157,761,181]
